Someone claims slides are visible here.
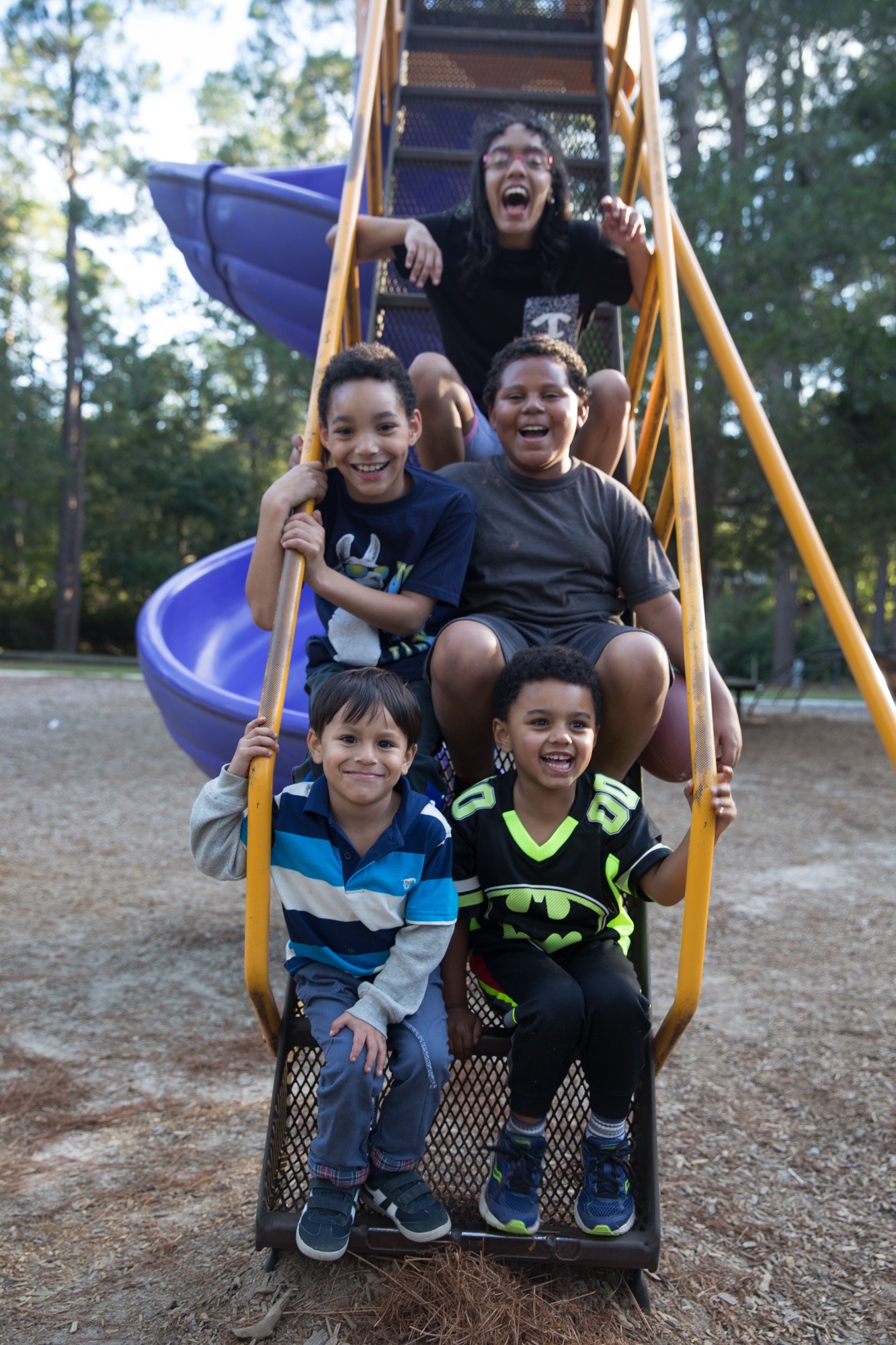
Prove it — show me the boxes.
[132,148,449,804]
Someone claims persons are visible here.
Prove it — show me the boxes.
[421,333,743,810]
[245,342,477,810]
[326,110,651,475]
[439,648,739,1239]
[189,668,457,1261]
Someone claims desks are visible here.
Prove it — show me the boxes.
[726,683,763,720]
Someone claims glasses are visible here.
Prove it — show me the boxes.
[482,151,552,171]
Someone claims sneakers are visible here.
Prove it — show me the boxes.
[359,1153,451,1243]
[573,1122,635,1236]
[478,1116,547,1236]
[295,1177,361,1262]
